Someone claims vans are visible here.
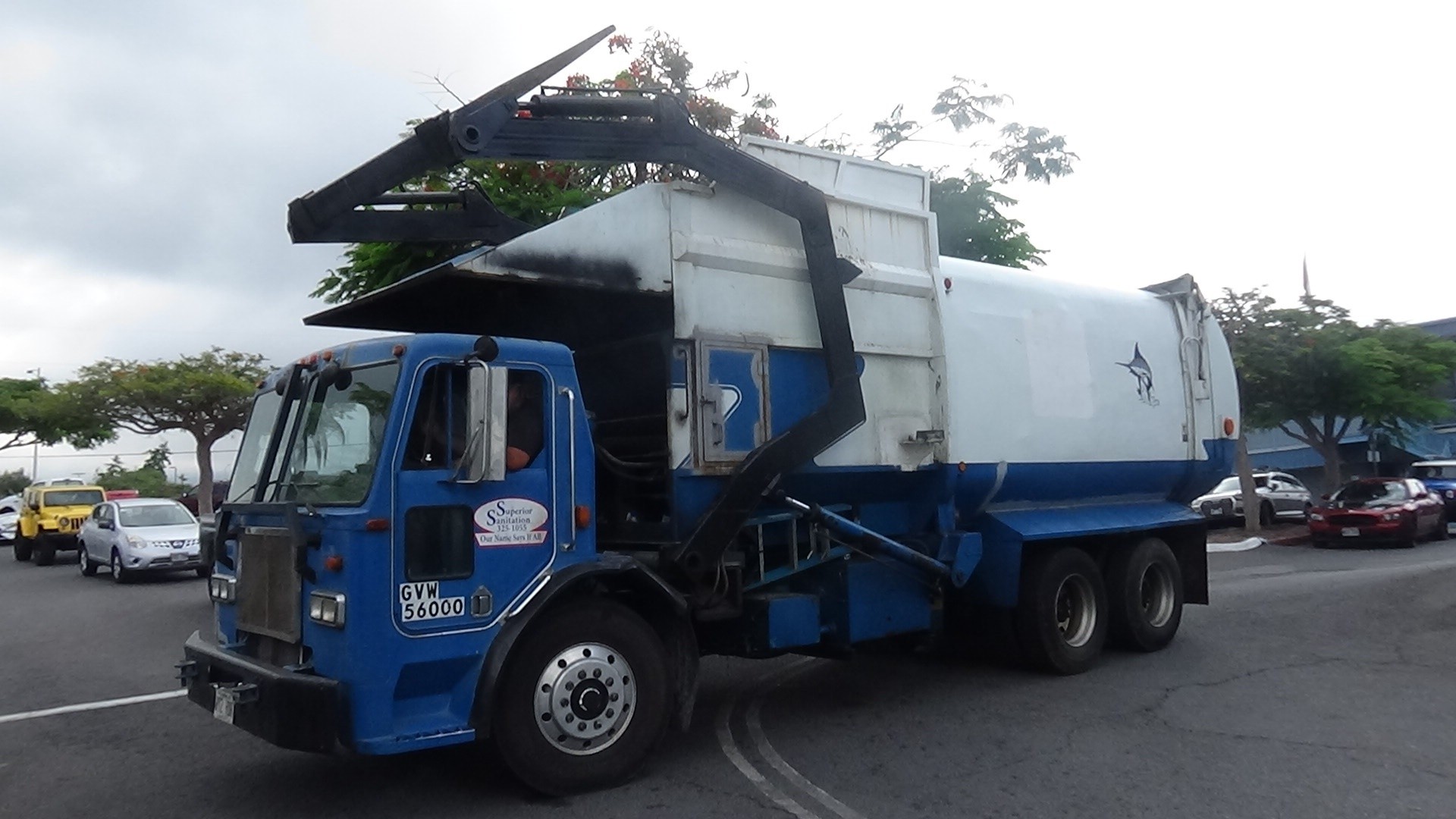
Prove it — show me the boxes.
[1406,459,1456,522]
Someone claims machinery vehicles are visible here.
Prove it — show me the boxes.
[172,23,1246,801]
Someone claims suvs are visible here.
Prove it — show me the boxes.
[13,484,109,566]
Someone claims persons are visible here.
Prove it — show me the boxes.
[433,367,541,470]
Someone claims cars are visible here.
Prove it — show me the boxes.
[174,480,229,519]
[0,478,143,545]
[77,497,204,586]
[1190,468,1314,529]
[1305,476,1449,549]
